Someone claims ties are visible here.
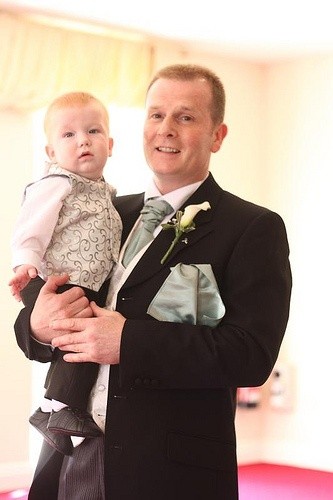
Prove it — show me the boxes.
[121,199,170,269]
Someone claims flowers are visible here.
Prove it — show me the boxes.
[160,201,211,264]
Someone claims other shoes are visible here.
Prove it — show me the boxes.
[46,406,103,438]
[29,407,73,456]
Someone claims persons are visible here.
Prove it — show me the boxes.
[14,63,292,500]
[8,92,123,456]
